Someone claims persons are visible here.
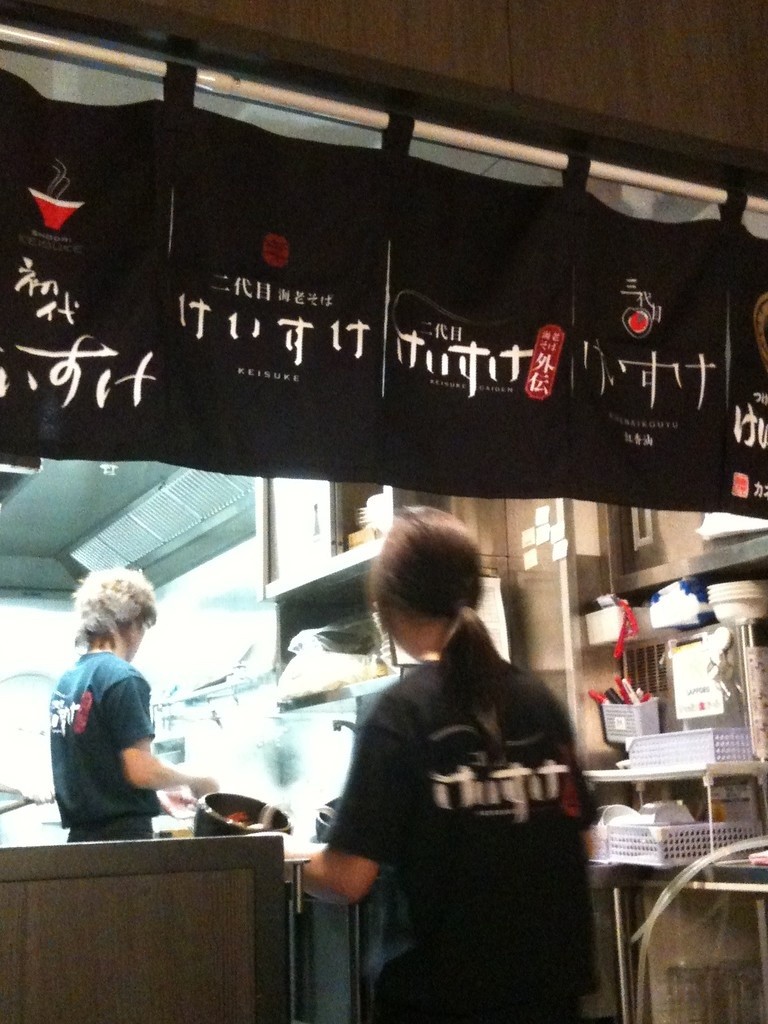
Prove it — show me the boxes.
[49,566,221,842]
[284,505,601,1024]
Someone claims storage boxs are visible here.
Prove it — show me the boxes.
[624,727,755,770]
[600,696,661,745]
[589,822,763,869]
[584,606,680,646]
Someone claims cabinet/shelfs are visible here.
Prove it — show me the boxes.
[252,477,418,716]
[554,500,766,893]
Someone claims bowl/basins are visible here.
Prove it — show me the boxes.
[705,577,768,626]
[597,804,641,827]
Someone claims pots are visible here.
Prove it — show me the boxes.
[193,790,293,848]
